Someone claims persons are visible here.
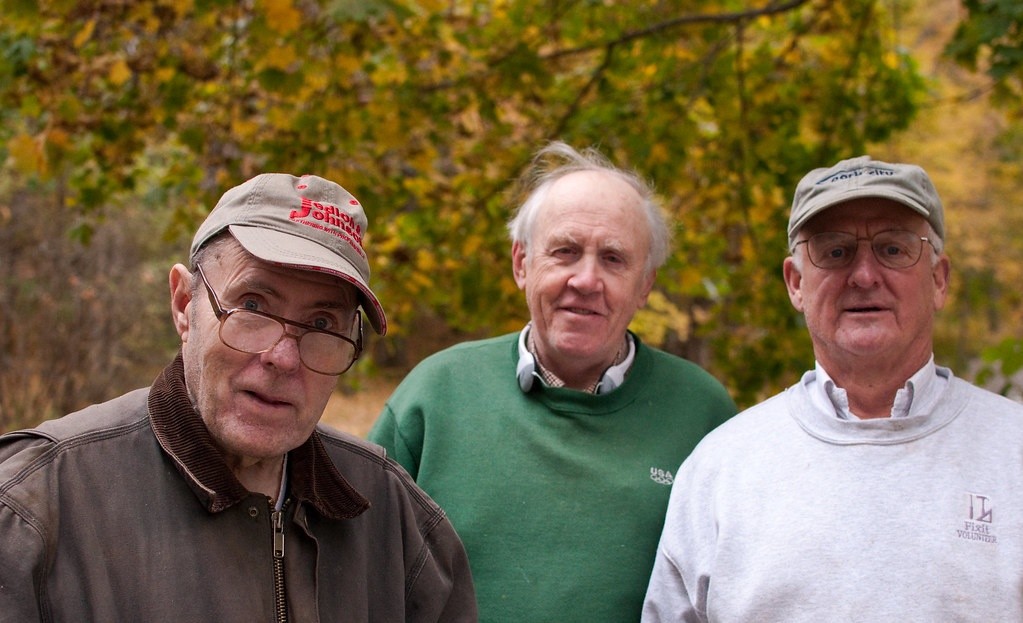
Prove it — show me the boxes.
[0,173,479,623]
[641,155,1023,623]
[365,141,740,623]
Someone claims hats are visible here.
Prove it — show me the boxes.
[787,156,944,255]
[190,173,387,337]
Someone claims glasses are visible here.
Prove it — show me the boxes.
[194,263,363,377]
[793,230,939,270]
